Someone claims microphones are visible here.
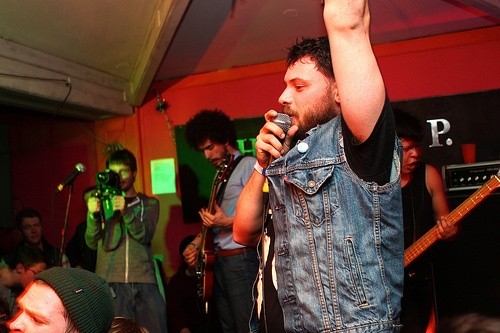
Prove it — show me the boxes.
[262,112,291,206]
[53,163,86,195]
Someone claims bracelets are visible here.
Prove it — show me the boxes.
[254,161,266,175]
[191,242,200,251]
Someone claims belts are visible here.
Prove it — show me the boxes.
[214,246,255,257]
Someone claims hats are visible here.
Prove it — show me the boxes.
[32,267,114,333]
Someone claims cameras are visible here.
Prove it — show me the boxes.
[90,169,121,201]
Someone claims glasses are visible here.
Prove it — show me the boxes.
[403,143,421,152]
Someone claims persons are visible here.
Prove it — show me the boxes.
[84,148,167,333]
[184,109,269,333]
[233,0,404,333]
[0,186,221,333]
[393,108,458,333]
[9,267,115,333]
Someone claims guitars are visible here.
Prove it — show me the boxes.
[400,170,500,269]
[193,153,237,303]
